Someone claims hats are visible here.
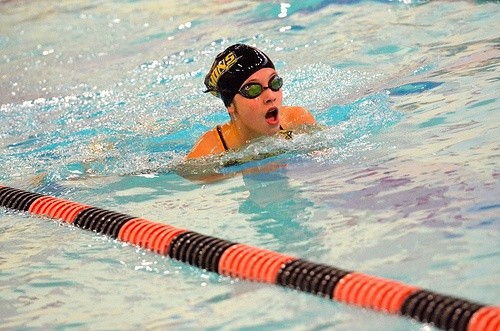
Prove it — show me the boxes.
[204,44,275,106]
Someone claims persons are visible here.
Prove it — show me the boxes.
[185,43,317,159]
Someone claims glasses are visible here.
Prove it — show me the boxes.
[238,75,282,100]
